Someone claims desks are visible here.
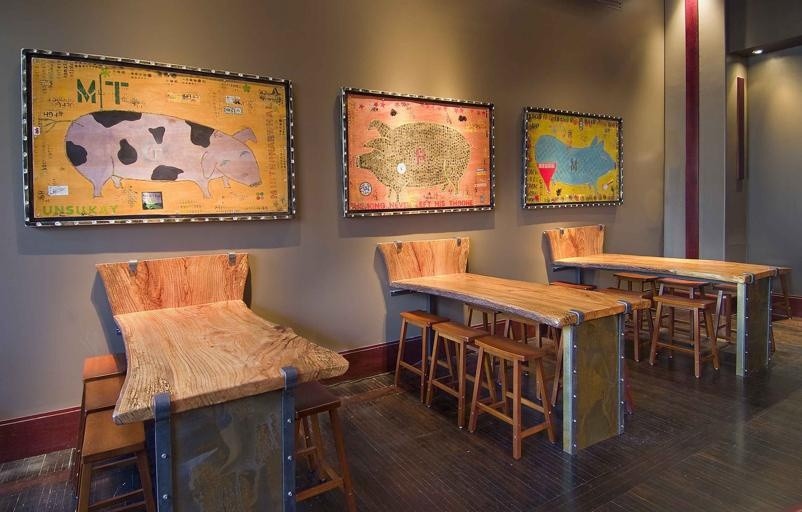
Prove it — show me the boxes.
[545,225,779,377]
[377,236,652,456]
[96,253,350,512]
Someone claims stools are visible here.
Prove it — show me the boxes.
[393,268,792,459]
[74,352,156,512]
[294,381,356,512]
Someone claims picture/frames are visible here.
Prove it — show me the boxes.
[20,46,297,229]
[522,106,625,210]
[340,86,496,219]
